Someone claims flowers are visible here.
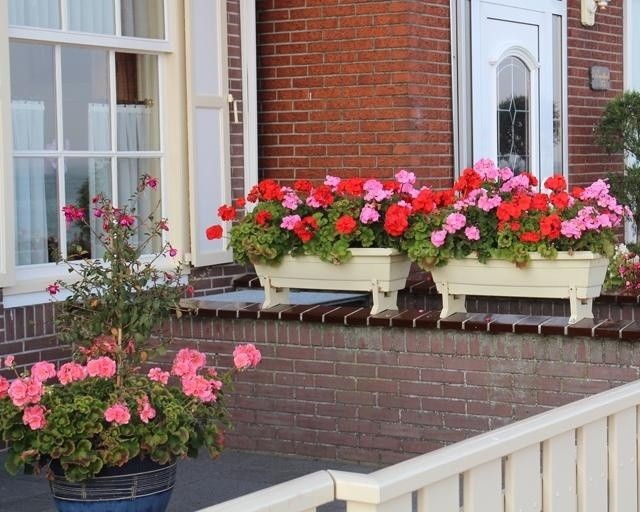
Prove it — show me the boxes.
[204,159,640,294]
[0,172,263,482]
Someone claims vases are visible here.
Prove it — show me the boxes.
[251,245,411,314]
[47,451,178,512]
[429,250,610,325]
